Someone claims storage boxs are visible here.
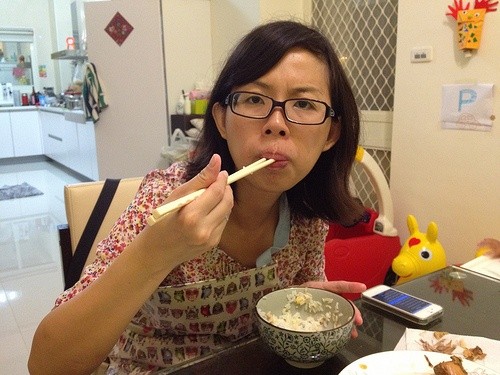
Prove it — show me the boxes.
[191,100,207,114]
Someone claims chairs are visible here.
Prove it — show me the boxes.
[57,177,143,293]
[323,147,403,301]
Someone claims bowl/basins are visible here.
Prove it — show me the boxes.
[254,287,356,369]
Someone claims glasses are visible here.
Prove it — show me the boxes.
[224,91,336,125]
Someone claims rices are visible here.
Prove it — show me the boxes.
[257,287,352,333]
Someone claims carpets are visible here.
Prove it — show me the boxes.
[0,182,44,200]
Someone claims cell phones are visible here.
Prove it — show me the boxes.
[360,283,444,326]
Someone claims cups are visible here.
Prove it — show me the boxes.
[14,90,20,106]
[22,93,28,106]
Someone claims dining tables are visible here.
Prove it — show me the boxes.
[152,266,500,375]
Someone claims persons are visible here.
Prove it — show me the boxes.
[28,21,367,375]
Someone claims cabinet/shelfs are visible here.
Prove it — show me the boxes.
[0,111,98,182]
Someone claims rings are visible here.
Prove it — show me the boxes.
[225,216,228,222]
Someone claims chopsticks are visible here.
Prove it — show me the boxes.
[145,157,275,227]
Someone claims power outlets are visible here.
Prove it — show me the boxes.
[412,47,432,62]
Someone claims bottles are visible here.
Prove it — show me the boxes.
[30,87,36,105]
[184,95,191,115]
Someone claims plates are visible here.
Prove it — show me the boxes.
[338,350,498,375]
